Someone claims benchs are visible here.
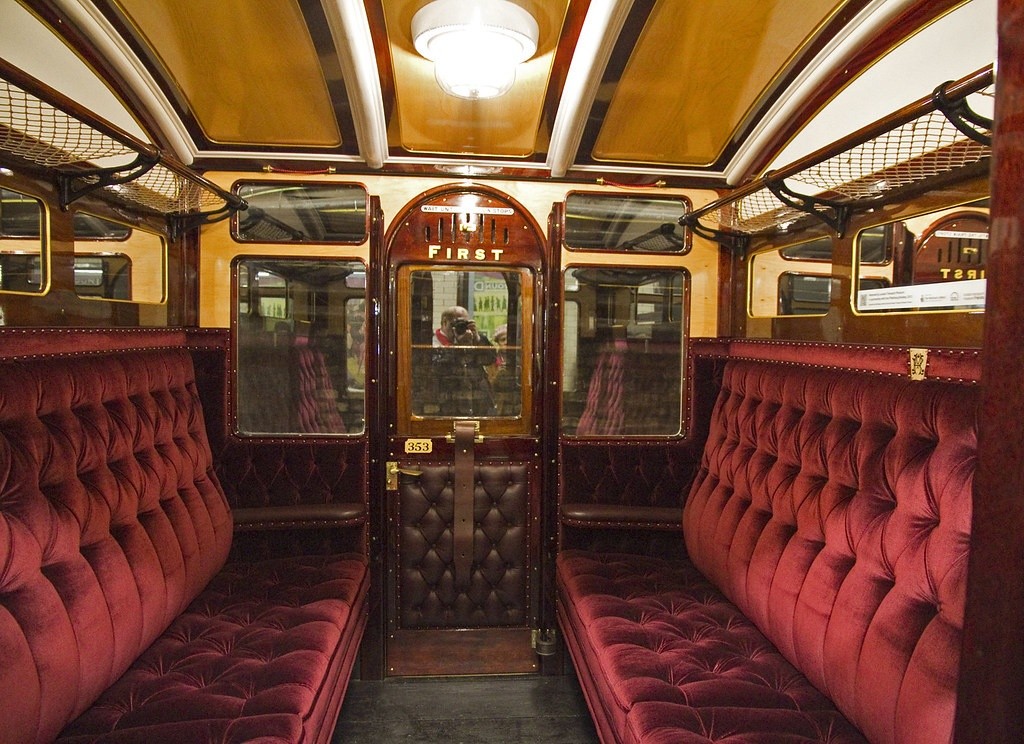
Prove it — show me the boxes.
[576,339,678,436]
[238,335,349,437]
[557,337,986,744]
[0,326,370,744]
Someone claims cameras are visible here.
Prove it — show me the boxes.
[451,317,469,335]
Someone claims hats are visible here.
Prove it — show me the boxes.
[493,324,507,343]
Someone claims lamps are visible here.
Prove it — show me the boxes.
[411,0,539,100]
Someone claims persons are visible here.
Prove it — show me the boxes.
[431,307,510,417]
